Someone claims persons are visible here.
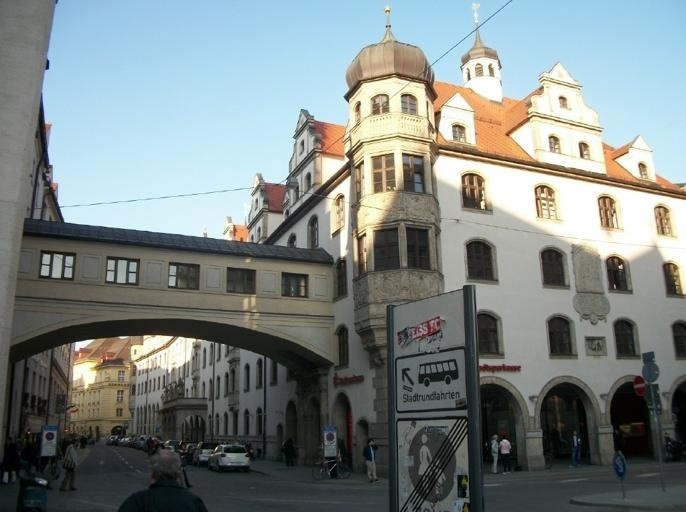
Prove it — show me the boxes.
[284,438,295,467]
[59,441,79,491]
[118,449,209,512]
[363,438,379,483]
[1,436,21,485]
[490,434,511,475]
[174,441,193,489]
[145,436,155,456]
[568,430,583,469]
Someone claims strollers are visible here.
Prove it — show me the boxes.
[663,432,686,463]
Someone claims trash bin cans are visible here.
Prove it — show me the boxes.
[18,476,47,512]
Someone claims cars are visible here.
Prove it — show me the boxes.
[103,434,254,475]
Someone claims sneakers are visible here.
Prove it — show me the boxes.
[59,488,77,492]
[491,472,512,475]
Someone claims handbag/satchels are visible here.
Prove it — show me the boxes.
[62,460,76,470]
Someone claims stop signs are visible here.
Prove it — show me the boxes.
[633,374,648,398]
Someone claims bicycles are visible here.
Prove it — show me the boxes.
[309,448,354,482]
